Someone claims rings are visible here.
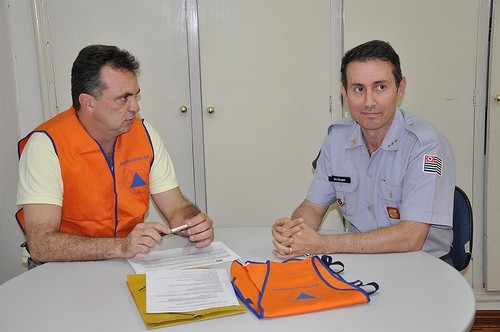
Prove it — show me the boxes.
[289,248,293,255]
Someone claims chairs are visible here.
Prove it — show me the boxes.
[450,185,473,270]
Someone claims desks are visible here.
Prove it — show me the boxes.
[0,227,476,332]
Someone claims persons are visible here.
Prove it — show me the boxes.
[271,39,455,267]
[15,45,214,271]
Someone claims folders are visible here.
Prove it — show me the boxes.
[125,275,247,330]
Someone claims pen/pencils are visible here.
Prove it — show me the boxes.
[160,224,190,237]
